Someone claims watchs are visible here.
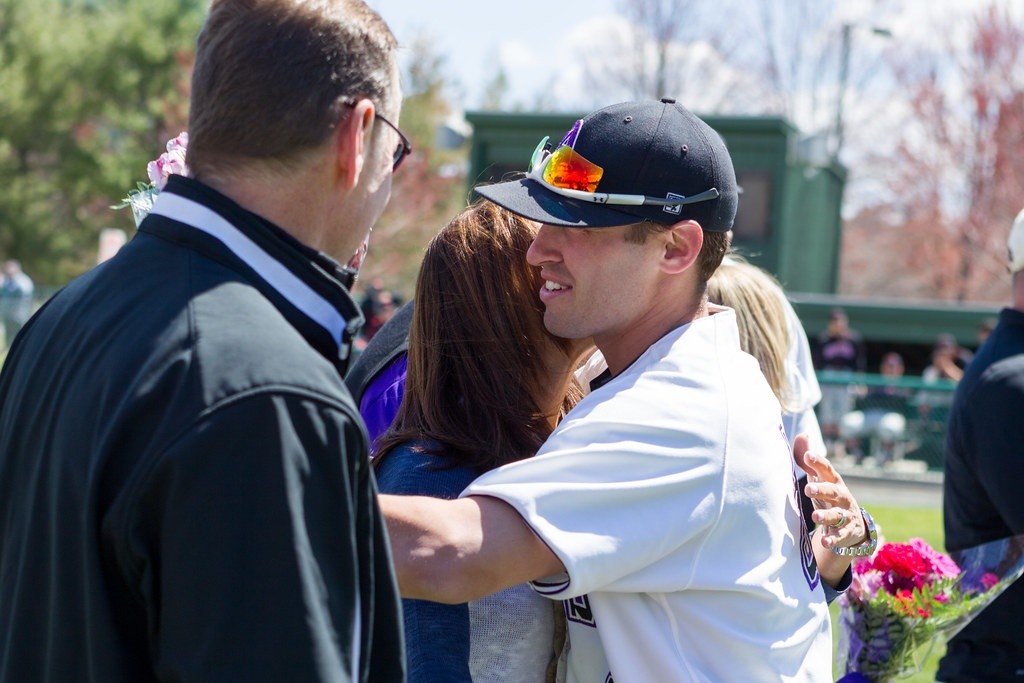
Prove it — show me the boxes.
[821,507,877,556]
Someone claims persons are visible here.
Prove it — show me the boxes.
[347,254,880,605]
[810,309,1002,471]
[377,99,834,683]
[936,207,1024,683]
[0,259,34,347]
[0,0,407,683]
[367,197,878,683]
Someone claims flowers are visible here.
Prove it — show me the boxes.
[836,538,999,683]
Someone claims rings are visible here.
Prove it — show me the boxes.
[829,510,846,528]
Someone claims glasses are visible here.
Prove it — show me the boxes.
[525,136,718,208]
[350,100,411,173]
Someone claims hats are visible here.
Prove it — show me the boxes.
[474,98,738,234]
[1004,205,1024,272]
